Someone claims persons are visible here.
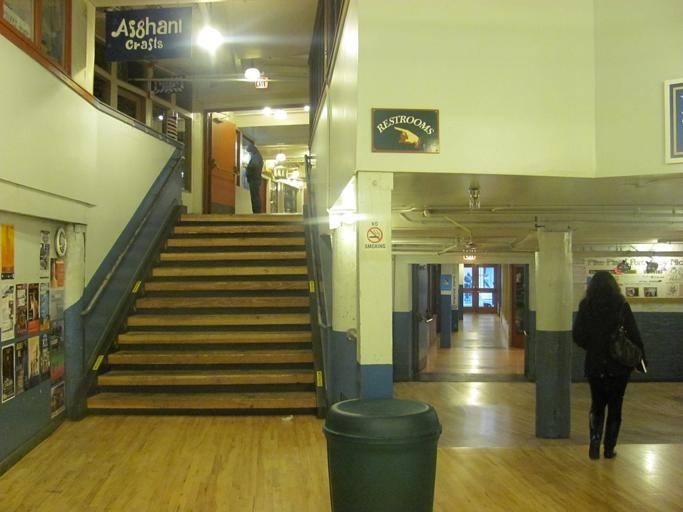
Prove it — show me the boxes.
[572,269,648,461]
[242,142,263,214]
[29,289,39,319]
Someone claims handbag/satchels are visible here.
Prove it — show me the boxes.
[611,325,640,372]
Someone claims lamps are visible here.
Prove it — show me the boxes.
[244,60,260,82]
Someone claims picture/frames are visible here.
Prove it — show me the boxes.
[0,0,72,77]
[664,78,683,165]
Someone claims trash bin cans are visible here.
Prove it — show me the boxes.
[322,396,441,512]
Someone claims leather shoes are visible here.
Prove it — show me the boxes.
[603,446,618,460]
[587,437,601,463]
